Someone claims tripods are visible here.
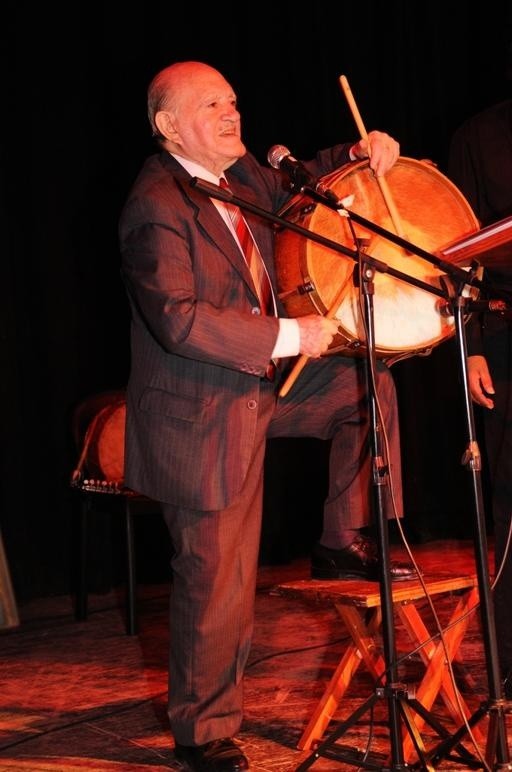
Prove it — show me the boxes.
[297,282,485,772]
[408,299,512,772]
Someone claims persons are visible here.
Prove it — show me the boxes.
[446,97,511,700]
[117,58,405,771]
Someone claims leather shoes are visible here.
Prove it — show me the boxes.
[311,540,425,582]
[173,737,249,772]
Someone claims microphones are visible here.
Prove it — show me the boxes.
[268,145,340,204]
[440,301,487,318]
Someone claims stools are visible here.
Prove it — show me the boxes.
[268,574,494,765]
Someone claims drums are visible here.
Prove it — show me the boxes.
[72,396,140,497]
[272,155,484,366]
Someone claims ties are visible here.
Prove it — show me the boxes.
[219,177,276,382]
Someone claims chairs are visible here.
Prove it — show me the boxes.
[72,391,150,636]
